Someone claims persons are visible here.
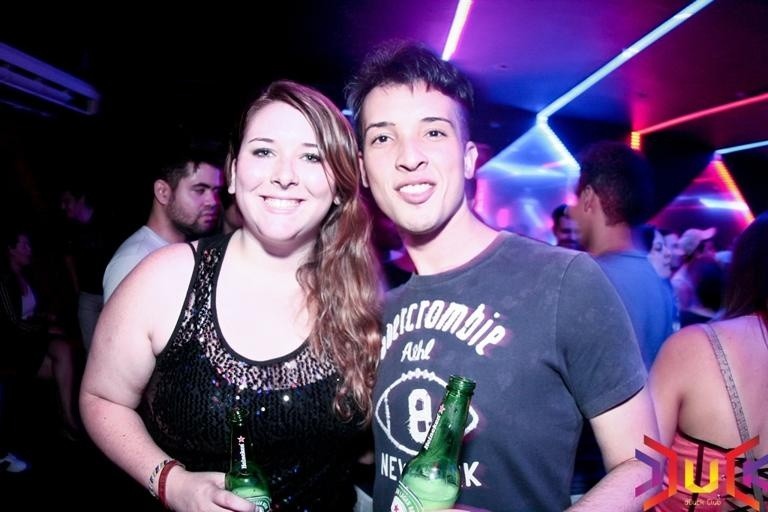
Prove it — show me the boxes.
[219,139,719,370]
[349,39,663,511]
[66,82,388,511]
[53,184,112,350]
[0,234,83,477]
[635,210,766,509]
[99,135,221,311]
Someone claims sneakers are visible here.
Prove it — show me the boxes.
[1,453,26,472]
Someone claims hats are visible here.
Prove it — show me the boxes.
[678,228,716,255]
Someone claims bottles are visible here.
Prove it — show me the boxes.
[224,408,273,512]
[387,373,476,512]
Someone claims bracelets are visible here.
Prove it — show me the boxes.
[140,456,185,506]
